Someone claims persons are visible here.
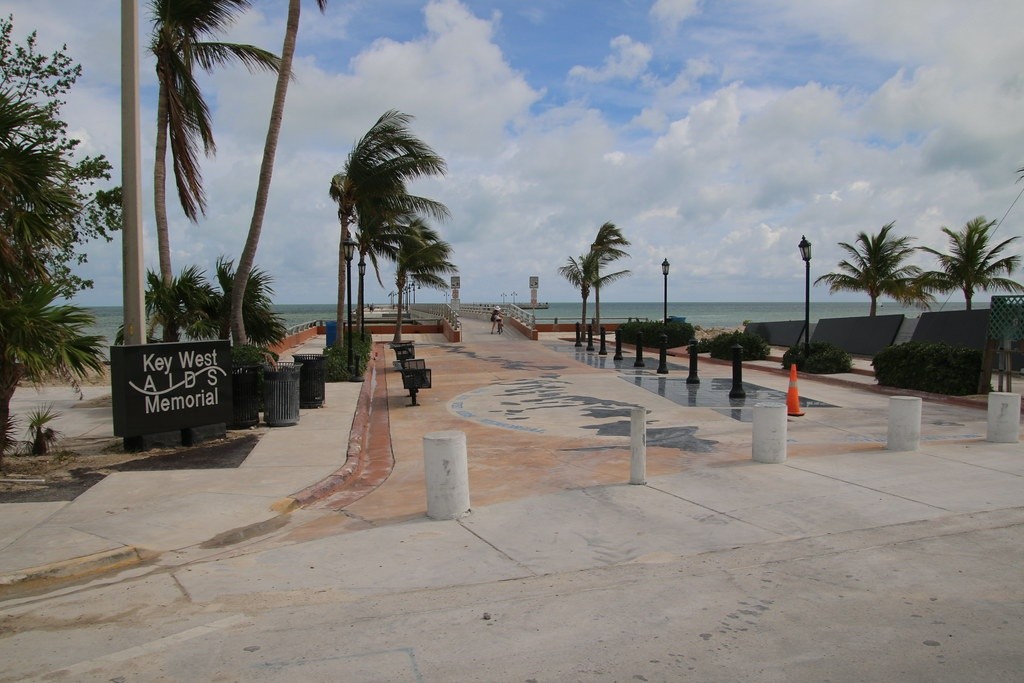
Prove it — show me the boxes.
[491,306,504,335]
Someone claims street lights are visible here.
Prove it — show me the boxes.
[798,235,812,343]
[357,256,367,341]
[444,292,449,303]
[501,292,507,303]
[662,257,670,326]
[342,233,356,368]
[511,291,517,302]
[387,282,421,312]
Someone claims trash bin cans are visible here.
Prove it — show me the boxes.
[268,361,305,427]
[292,352,330,409]
[229,364,263,429]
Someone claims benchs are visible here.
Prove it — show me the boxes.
[388,342,431,406]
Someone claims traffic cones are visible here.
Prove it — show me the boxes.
[785,363,805,417]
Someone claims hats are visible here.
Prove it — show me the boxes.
[494,306,501,310]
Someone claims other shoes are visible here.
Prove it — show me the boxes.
[491,332,493,334]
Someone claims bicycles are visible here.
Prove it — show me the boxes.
[496,319,503,335]
